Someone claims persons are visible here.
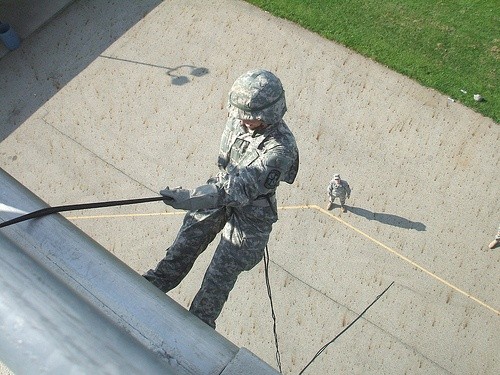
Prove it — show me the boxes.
[326,173,351,213]
[142,69,299,331]
[488,230,500,249]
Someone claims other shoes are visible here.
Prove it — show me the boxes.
[341,206,347,213]
[327,204,333,210]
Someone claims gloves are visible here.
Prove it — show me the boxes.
[160,184,219,211]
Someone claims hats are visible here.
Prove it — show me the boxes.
[334,174,340,180]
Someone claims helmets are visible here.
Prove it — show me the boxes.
[227,70,287,126]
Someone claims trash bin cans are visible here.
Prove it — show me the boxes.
[0,22,22,51]
